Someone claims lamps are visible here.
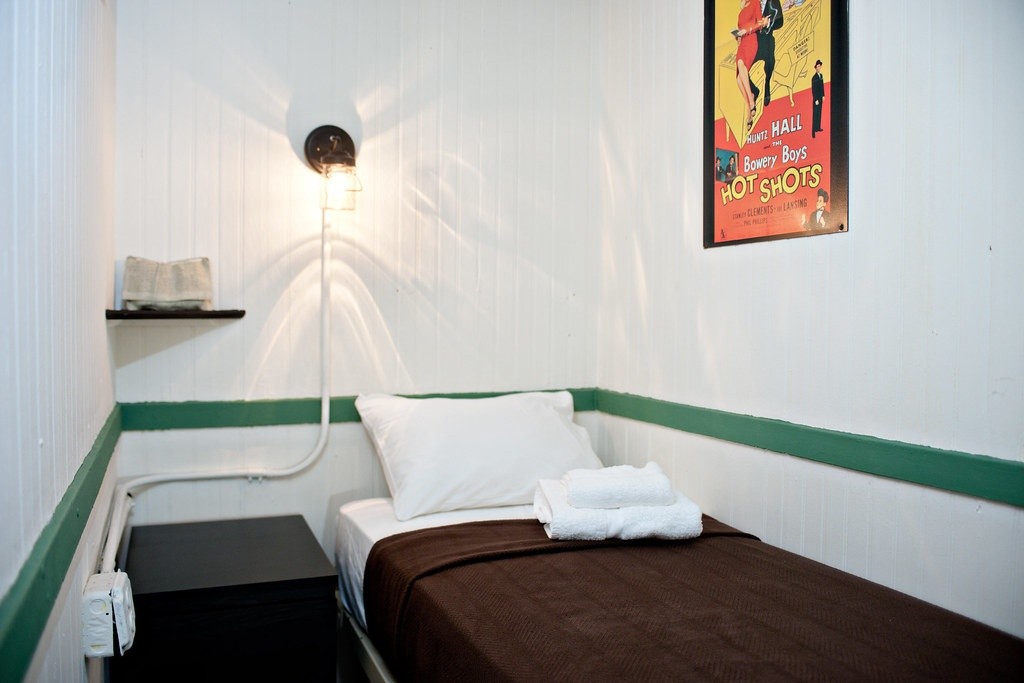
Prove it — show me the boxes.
[305,125,358,210]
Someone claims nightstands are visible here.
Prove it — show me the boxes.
[106,514,342,683]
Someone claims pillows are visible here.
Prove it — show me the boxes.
[355,391,604,520]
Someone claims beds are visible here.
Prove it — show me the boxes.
[336,498,1024,683]
